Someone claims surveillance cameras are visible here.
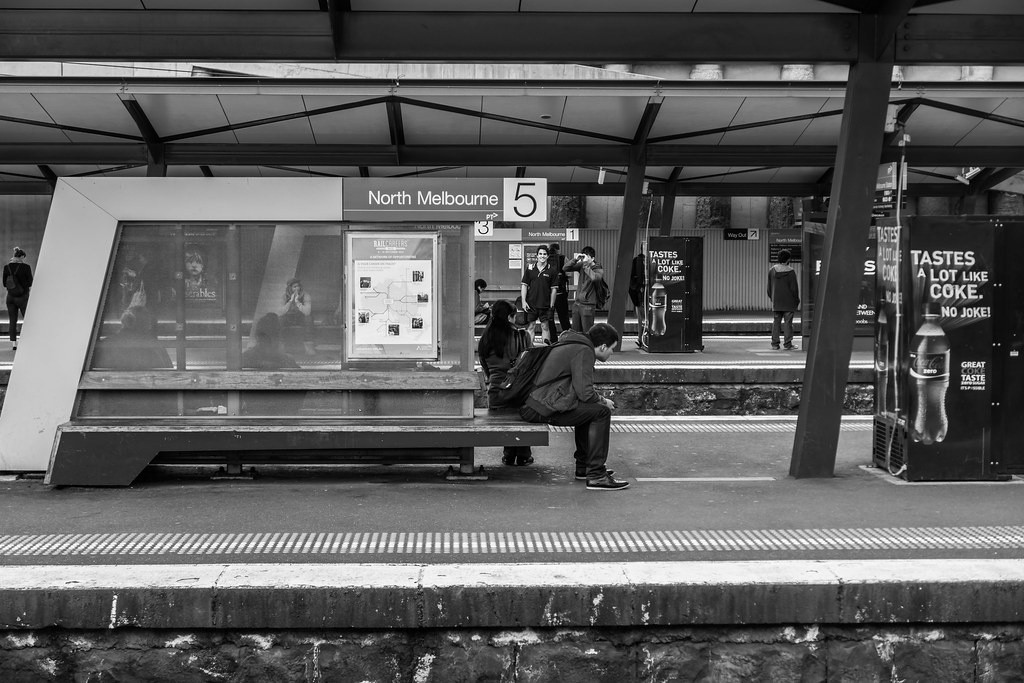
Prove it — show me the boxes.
[598,169,606,185]
[954,175,970,186]
[642,181,649,194]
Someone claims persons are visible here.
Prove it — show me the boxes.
[183,245,216,304]
[628,243,646,348]
[240,312,306,416]
[520,245,559,345]
[562,246,604,334]
[282,278,312,355]
[767,248,801,351]
[521,323,630,490]
[3,247,33,350]
[474,279,491,336]
[92,307,173,415]
[478,299,535,466]
[547,243,572,344]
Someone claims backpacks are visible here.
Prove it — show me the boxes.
[499,340,595,402]
[595,276,610,309]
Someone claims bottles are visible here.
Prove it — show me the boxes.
[875,295,889,417]
[649,274,667,336]
[912,302,950,444]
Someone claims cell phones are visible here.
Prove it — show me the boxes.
[613,405,618,409]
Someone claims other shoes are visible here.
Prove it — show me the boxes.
[773,344,780,349]
[501,455,514,465]
[517,456,534,465]
[784,342,798,349]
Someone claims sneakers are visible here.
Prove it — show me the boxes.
[586,474,630,490]
[575,469,615,479]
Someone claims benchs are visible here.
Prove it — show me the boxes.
[43,406,550,486]
